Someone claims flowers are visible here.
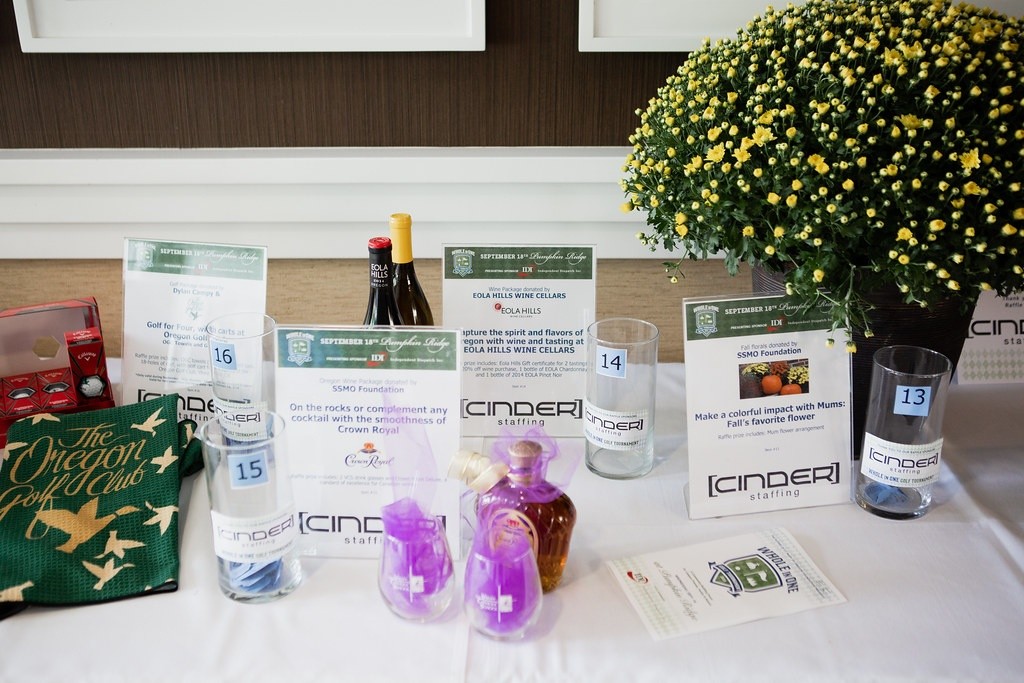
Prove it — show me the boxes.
[619,0,1024,354]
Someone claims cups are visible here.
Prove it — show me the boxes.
[381,504,456,622]
[462,543,544,637]
[585,317,657,479]
[205,314,277,445]
[198,408,304,601]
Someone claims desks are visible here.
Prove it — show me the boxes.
[0,355,1023,682]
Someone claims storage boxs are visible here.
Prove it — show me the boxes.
[0,296,115,450]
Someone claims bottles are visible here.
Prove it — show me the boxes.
[855,341,952,520]
[477,441,577,594]
[360,237,406,329]
[389,212,436,329]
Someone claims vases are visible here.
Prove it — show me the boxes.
[751,258,981,461]
